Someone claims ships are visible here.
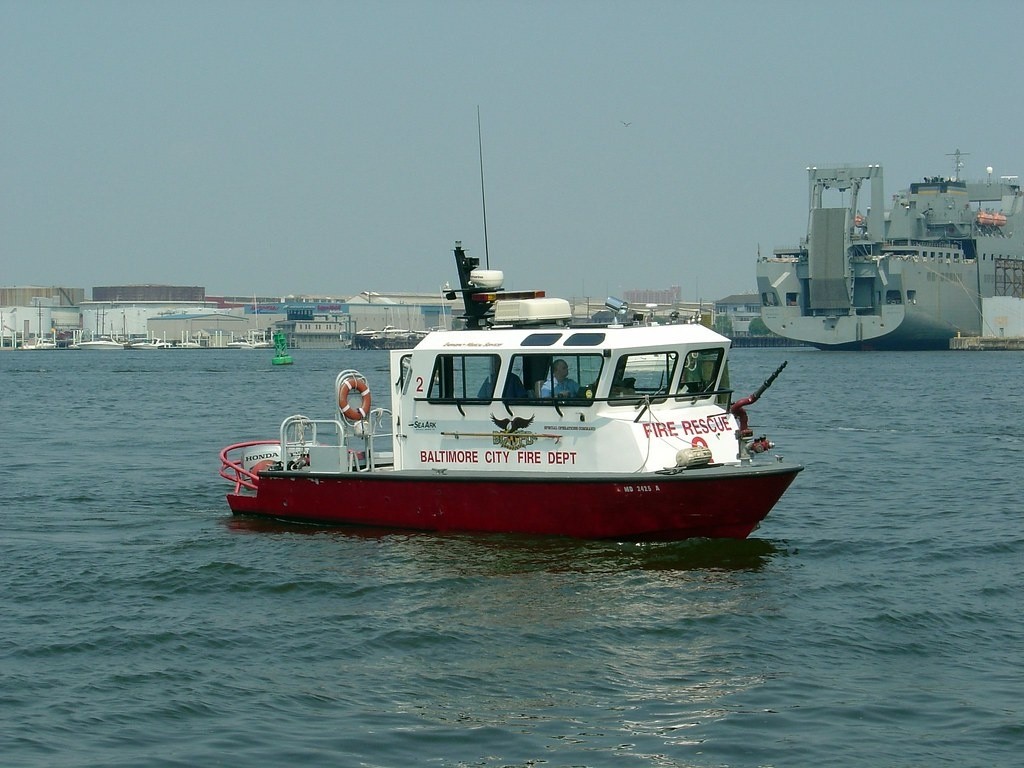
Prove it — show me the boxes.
[754,148,1024,352]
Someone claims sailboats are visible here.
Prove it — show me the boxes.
[355,285,448,341]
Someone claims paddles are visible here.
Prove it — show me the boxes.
[337,378,373,420]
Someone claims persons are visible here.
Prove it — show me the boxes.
[477,356,581,399]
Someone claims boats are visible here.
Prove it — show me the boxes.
[18,305,275,351]
[218,104,804,543]
[271,326,293,365]
